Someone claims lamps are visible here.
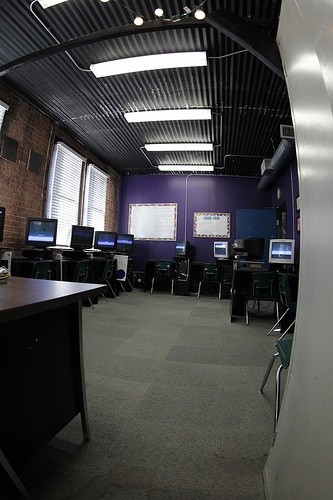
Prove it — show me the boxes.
[124,108,215,171]
[30,0,207,79]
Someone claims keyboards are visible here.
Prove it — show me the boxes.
[212,262,233,266]
[238,267,269,272]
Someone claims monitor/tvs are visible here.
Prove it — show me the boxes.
[175,241,196,256]
[116,233,134,252]
[234,236,266,267]
[213,242,230,261]
[70,226,94,251]
[25,217,58,251]
[0,207,6,241]
[268,239,296,269]
[94,231,118,252]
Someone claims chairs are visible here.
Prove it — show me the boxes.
[31,258,298,433]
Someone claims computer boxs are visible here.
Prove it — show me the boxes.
[172,256,192,262]
[93,252,118,257]
[113,252,132,259]
[62,251,90,258]
[22,249,54,258]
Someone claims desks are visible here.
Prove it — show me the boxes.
[0,276,108,475]
